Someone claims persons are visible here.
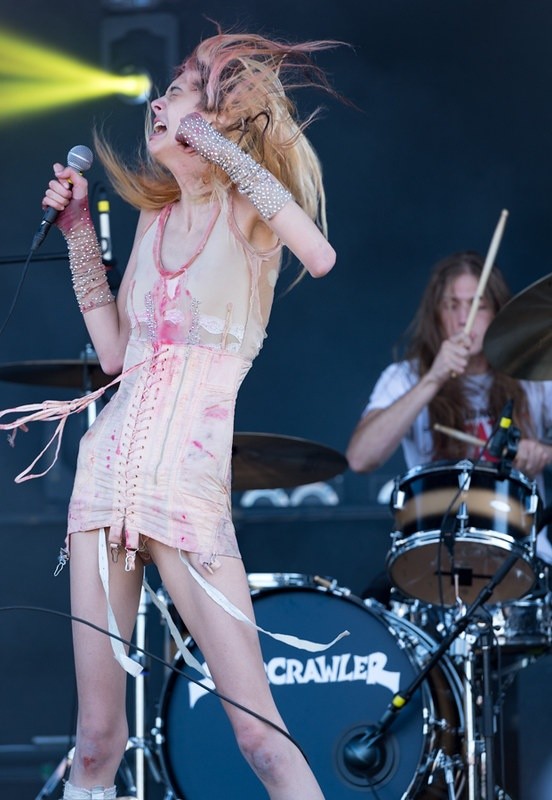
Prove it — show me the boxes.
[43,32,338,799]
[347,253,552,800]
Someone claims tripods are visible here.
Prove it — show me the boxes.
[124,589,164,800]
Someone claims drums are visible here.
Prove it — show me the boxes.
[387,455,543,607]
[153,571,476,800]
[389,550,551,678]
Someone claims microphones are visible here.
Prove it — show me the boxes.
[31,144,93,254]
[488,402,513,457]
[97,189,114,269]
[344,732,381,772]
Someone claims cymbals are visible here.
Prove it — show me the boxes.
[230,431,349,492]
[1,357,123,390]
[481,270,551,381]
